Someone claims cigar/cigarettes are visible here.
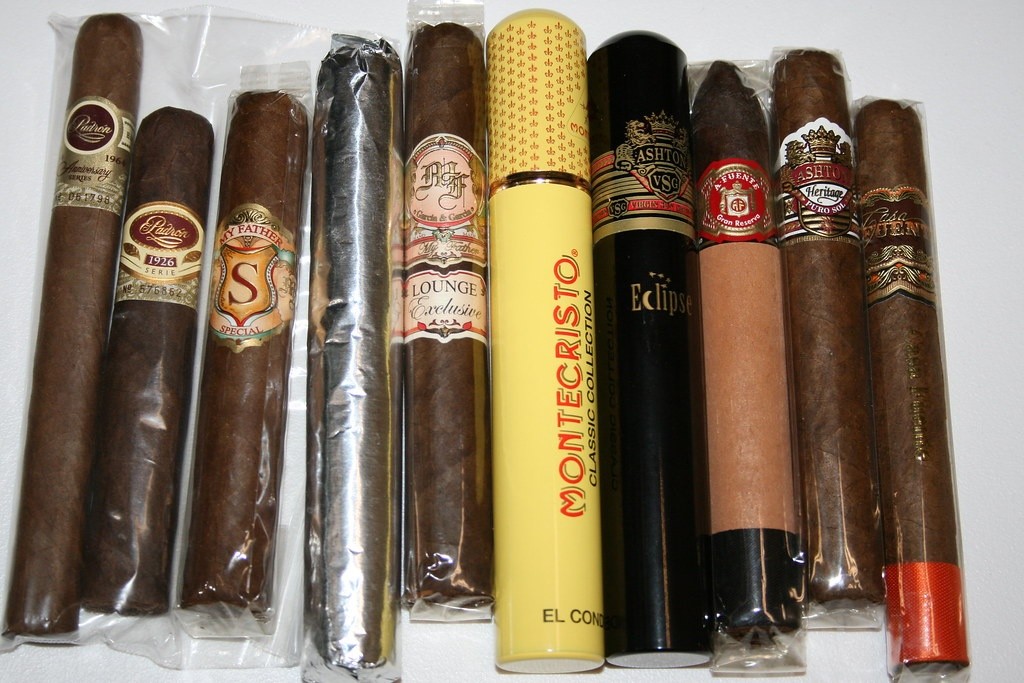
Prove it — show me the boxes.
[4,13,970,676]
